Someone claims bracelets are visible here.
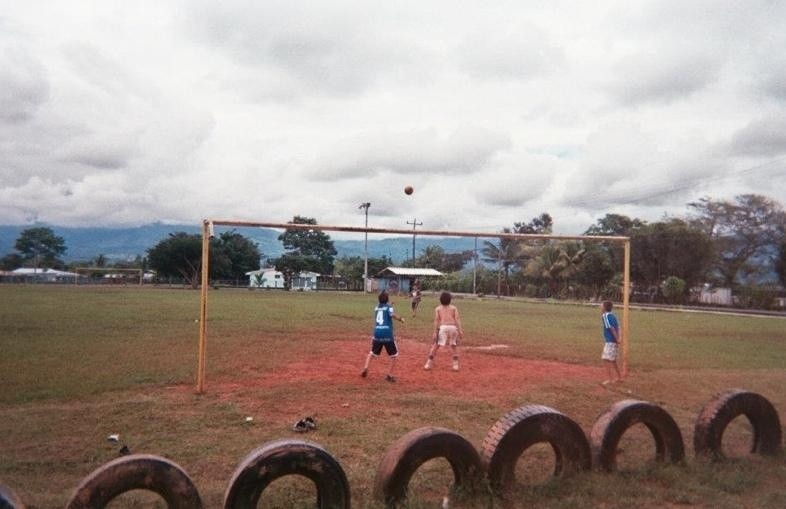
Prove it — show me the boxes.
[399,317,402,321]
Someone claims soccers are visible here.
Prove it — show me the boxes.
[405,186,413,195]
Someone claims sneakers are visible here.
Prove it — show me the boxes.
[424,363,459,371]
[602,378,624,386]
[362,368,396,383]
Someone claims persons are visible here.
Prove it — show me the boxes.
[408,284,422,318]
[424,292,464,372]
[600,300,622,386]
[361,289,406,383]
